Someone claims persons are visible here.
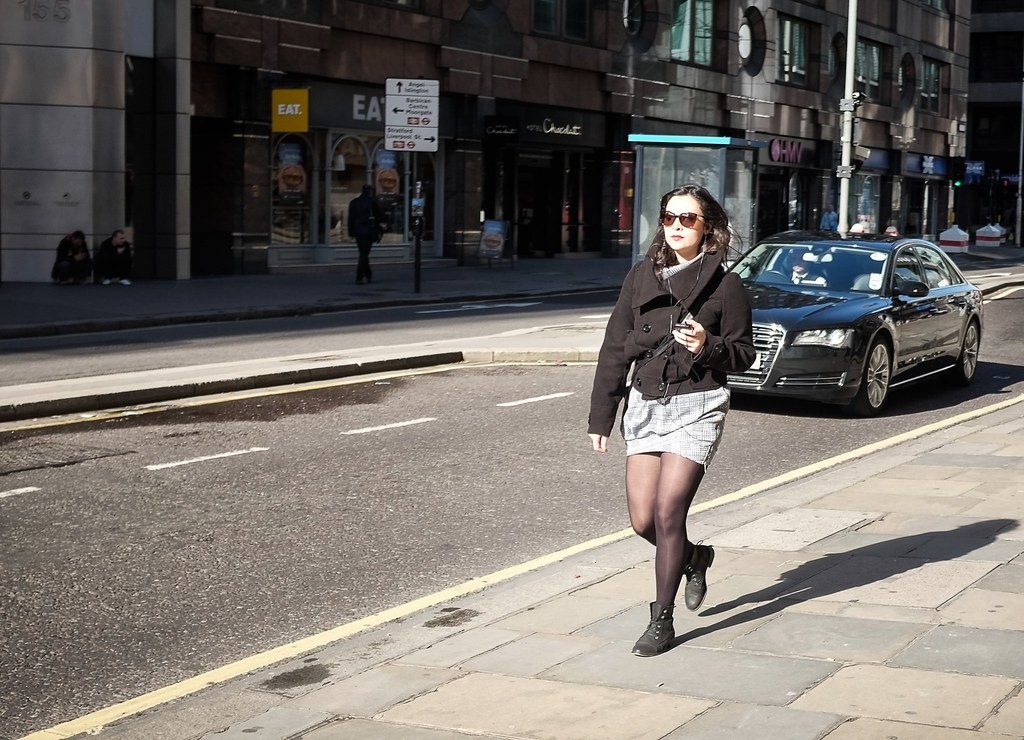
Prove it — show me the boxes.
[347,185,387,285]
[51,230,93,285]
[93,230,133,285]
[588,184,756,656]
[787,252,827,287]
[819,204,838,231]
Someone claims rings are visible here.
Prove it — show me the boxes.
[686,341,688,348]
[686,335,687,341]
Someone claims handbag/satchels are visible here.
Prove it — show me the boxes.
[368,216,384,243]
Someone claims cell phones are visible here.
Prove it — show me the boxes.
[675,324,691,332]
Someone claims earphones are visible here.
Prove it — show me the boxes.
[705,225,710,230]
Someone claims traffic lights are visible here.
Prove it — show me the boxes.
[951,156,967,188]
[999,177,1009,200]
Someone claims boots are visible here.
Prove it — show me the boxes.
[631,602,676,657]
[683,540,714,612]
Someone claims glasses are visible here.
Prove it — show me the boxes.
[660,211,705,228]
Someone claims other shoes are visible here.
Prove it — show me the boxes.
[119,279,132,285]
[102,279,112,284]
[355,280,365,284]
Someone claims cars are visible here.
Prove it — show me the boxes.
[723,228,984,419]
